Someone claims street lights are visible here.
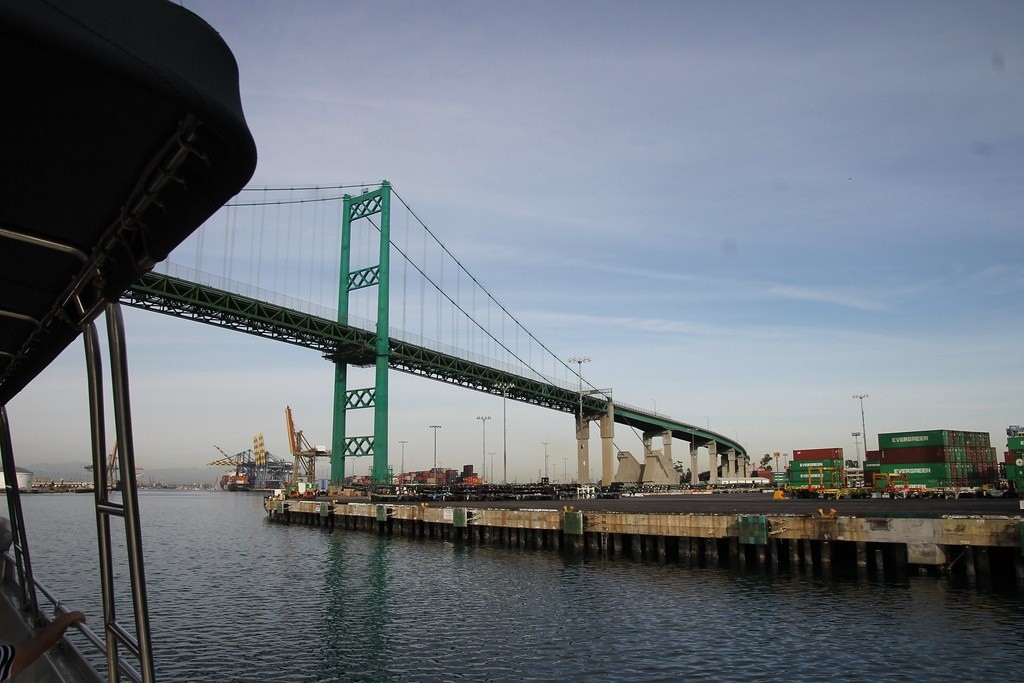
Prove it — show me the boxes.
[567,356,592,496]
[563,457,569,482]
[399,441,407,473]
[541,442,550,481]
[851,393,871,467]
[350,458,357,475]
[493,382,515,484]
[429,426,442,484]
[551,463,558,480]
[476,416,492,483]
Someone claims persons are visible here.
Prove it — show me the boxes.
[0,611,86,683]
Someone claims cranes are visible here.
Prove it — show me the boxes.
[207,405,333,490]
[84,441,118,485]
